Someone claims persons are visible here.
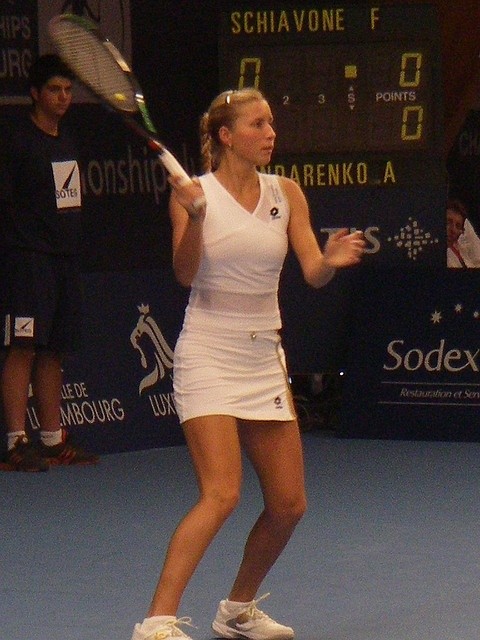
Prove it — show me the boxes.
[1,52,104,474]
[129,85,368,640]
[446,195,478,268]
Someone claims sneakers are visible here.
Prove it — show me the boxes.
[130,615,194,639]
[37,437,98,466]
[210,593,295,640]
[2,435,50,473]
